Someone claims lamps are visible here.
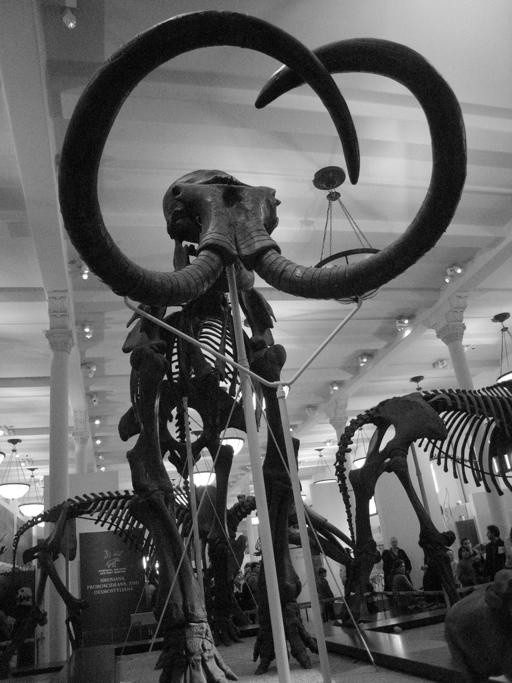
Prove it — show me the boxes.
[0,438,47,529]
[307,165,391,307]
[313,425,375,489]
[491,311,512,384]
[179,428,248,489]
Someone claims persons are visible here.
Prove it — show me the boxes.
[482,523,506,582]
[6,587,48,670]
[390,558,420,606]
[315,566,336,621]
[381,537,411,593]
[455,545,478,587]
[460,537,484,566]
